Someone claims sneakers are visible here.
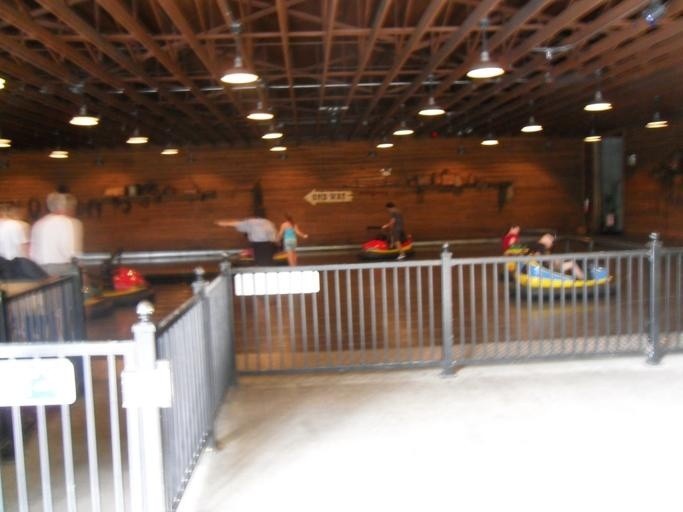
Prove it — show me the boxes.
[396,255,405,260]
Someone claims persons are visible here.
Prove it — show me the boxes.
[382,203,408,261]
[2,192,86,340]
[502,225,585,281]
[278,212,308,264]
[214,208,278,264]
